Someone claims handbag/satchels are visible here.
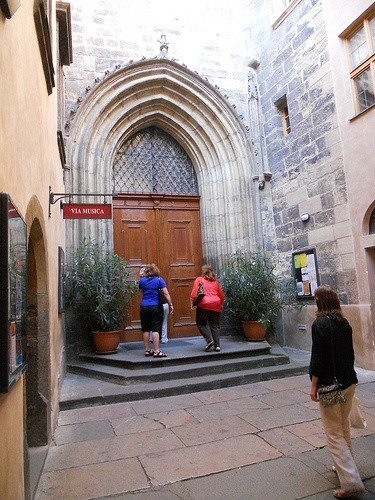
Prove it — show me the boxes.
[193,277,205,306]
[158,277,167,304]
[349,393,367,429]
[316,382,347,409]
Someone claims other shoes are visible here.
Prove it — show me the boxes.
[161,337,168,344]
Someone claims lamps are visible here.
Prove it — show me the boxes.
[301,214,310,222]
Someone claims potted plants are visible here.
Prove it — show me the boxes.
[209,248,282,340]
[65,236,136,352]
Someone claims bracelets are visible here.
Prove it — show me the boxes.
[169,305,173,306]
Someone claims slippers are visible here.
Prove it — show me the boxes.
[206,341,214,352]
[213,346,221,352]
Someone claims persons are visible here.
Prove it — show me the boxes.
[139,267,169,344]
[190,264,224,351]
[139,263,173,358]
[308,284,365,497]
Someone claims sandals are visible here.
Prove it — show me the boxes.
[333,486,365,498]
[145,349,154,356]
[153,350,167,357]
